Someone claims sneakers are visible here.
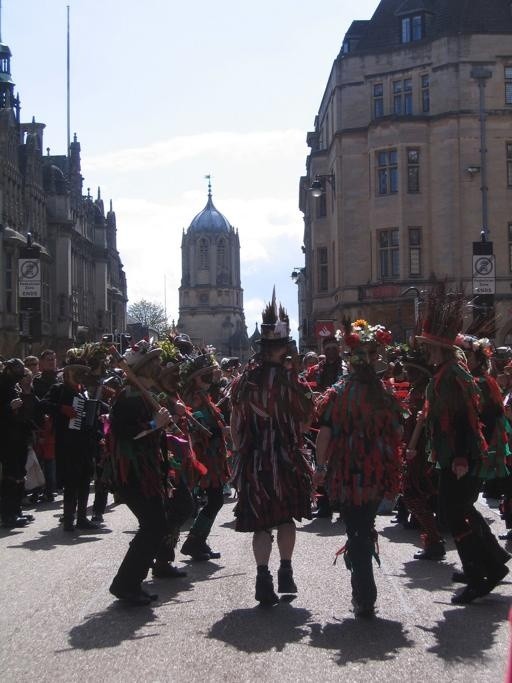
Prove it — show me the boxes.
[279,568,298,593]
[311,510,332,518]
[391,513,511,602]
[255,575,278,601]
[110,544,220,605]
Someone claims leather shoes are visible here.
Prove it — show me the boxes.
[0,491,104,531]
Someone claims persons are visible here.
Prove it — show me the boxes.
[0,273,511,618]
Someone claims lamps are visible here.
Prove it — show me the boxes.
[311,174,334,197]
[291,268,302,280]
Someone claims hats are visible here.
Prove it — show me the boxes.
[255,321,512,370]
[65,333,239,372]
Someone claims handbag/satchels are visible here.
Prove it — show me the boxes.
[24,446,46,489]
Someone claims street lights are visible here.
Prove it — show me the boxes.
[469,64,499,324]
